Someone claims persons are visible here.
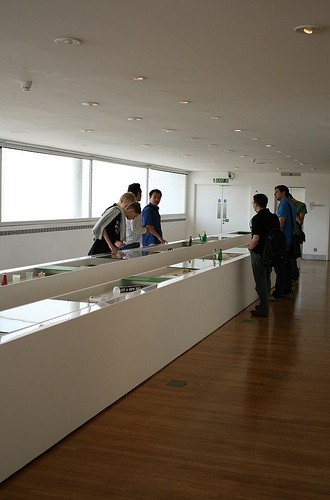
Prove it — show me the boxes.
[288,193,307,281]
[101,192,136,250]
[123,183,147,250]
[142,189,168,247]
[88,202,141,256]
[247,194,281,319]
[269,184,297,301]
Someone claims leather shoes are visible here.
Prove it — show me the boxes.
[255,305,269,311]
[250,310,269,317]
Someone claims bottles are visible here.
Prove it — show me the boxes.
[2,274,7,286]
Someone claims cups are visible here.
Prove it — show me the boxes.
[26,272,33,280]
[13,275,20,283]
[38,273,45,278]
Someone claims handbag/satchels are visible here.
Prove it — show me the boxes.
[296,230,305,244]
[293,233,301,259]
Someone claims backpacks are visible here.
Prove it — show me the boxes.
[262,233,275,261]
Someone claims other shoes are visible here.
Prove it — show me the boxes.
[282,293,293,298]
[269,295,283,302]
